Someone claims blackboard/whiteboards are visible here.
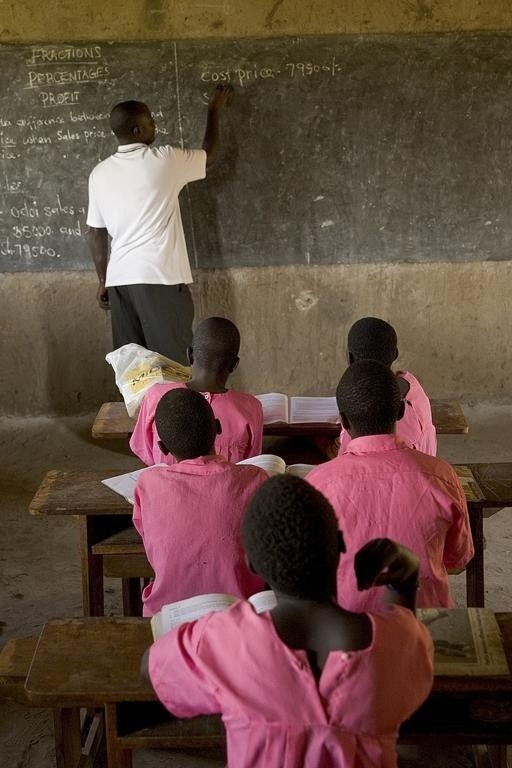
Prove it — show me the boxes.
[1,32,511,274]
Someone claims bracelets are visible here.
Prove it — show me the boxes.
[99,278,105,283]
[385,582,421,594]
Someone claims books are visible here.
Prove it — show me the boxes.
[101,462,169,507]
[253,392,342,425]
[235,453,316,480]
[149,589,277,644]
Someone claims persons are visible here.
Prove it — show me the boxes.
[86,84,230,367]
[129,317,264,466]
[315,316,438,465]
[131,388,270,617]
[138,472,436,768]
[302,359,475,615]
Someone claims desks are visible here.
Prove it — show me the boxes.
[26,608,512,768]
[91,399,469,464]
[28,463,512,615]
